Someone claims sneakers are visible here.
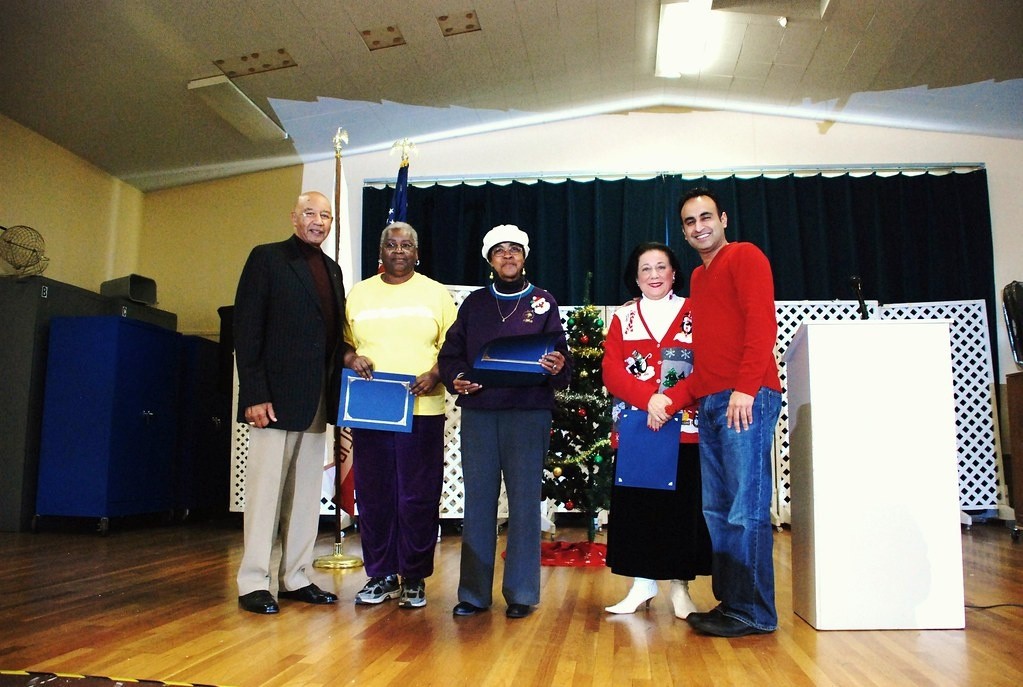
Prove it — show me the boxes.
[355,574,401,604]
[399,584,427,607]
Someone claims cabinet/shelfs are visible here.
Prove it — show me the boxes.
[0,274,359,533]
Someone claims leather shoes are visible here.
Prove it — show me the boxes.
[453,602,478,616]
[285,583,338,604]
[239,590,280,614]
[506,604,530,618]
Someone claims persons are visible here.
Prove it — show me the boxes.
[602,244,715,619]
[437,224,573,617]
[646,192,782,637]
[344,222,452,609]
[232,191,345,614]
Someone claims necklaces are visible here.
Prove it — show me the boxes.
[493,281,525,322]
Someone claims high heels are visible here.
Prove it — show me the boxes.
[605,577,657,613]
[670,579,697,619]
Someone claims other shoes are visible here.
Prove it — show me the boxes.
[686,608,776,637]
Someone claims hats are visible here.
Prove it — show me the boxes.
[482,225,530,264]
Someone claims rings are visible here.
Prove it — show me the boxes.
[464,389,469,395]
[247,421,256,426]
[551,364,557,370]
